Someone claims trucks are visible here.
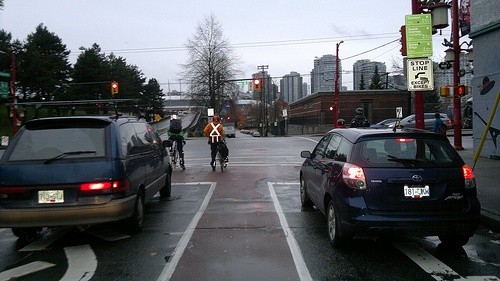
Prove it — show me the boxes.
[223,123,236,138]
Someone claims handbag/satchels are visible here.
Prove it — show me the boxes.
[438,124,448,134]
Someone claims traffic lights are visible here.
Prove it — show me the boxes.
[458,84,468,96]
[330,106,334,111]
[254,79,260,90]
[111,82,118,95]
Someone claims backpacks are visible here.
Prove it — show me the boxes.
[169,118,182,134]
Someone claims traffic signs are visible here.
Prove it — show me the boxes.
[406,59,435,91]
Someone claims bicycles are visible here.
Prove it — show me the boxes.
[168,136,185,165]
[215,138,228,172]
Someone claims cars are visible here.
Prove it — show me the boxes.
[369,119,400,128]
[240,129,260,137]
[300,127,481,248]
[178,111,187,116]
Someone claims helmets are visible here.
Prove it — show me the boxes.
[355,108,364,115]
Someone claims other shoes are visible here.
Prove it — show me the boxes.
[180,164,186,170]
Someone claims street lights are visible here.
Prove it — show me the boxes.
[334,41,344,128]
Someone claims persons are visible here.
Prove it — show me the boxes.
[350,108,370,128]
[204,117,229,165]
[336,119,346,128]
[167,115,186,169]
[433,114,443,132]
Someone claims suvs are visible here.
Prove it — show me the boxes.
[0,116,173,234]
[388,113,451,132]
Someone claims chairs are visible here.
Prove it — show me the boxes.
[384,140,401,156]
